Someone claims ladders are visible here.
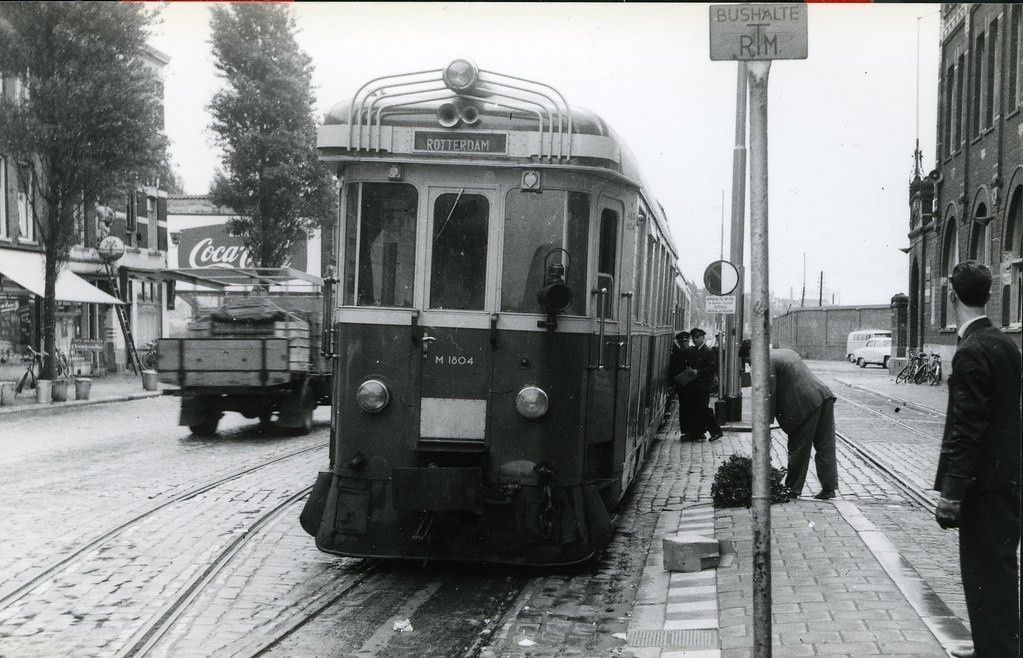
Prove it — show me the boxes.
[104,259,142,376]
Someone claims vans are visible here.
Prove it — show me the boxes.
[847,330,892,363]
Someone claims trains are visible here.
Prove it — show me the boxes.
[299,56,695,566]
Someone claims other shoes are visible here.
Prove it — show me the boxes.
[695,433,707,439]
[709,433,723,441]
[815,490,836,499]
[681,433,698,441]
[788,492,797,499]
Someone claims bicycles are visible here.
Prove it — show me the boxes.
[139,339,156,370]
[895,347,941,386]
[14,345,71,398]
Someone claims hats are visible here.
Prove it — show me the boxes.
[690,328,706,337]
[715,334,724,338]
[675,332,690,340]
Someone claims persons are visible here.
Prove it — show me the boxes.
[739,339,840,500]
[686,328,724,442]
[709,331,727,397]
[667,332,702,441]
[934,260,1023,658]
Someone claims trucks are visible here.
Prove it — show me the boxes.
[157,297,332,436]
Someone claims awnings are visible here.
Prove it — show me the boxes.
[0,247,125,309]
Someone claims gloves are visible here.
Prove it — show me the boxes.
[935,498,961,529]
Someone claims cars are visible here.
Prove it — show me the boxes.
[854,337,892,369]
